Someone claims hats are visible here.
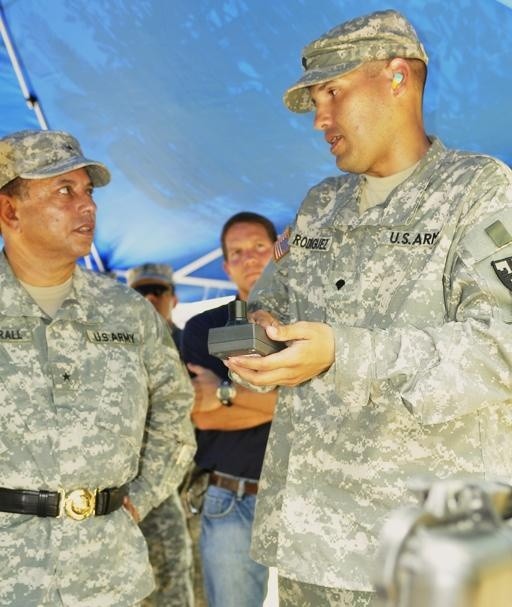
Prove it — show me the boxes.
[125,264,176,289]
[284,10,427,113]
[0,131,112,189]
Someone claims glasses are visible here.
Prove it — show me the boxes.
[134,284,168,300]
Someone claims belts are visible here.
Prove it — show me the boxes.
[0,482,127,521]
[208,473,258,495]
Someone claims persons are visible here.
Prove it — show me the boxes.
[127,262,210,607]
[223,9,512,607]
[133,490,195,607]
[0,130,198,607]
[180,212,280,607]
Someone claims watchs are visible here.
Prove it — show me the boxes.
[216,380,236,407]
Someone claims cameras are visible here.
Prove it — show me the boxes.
[208,300,287,360]
